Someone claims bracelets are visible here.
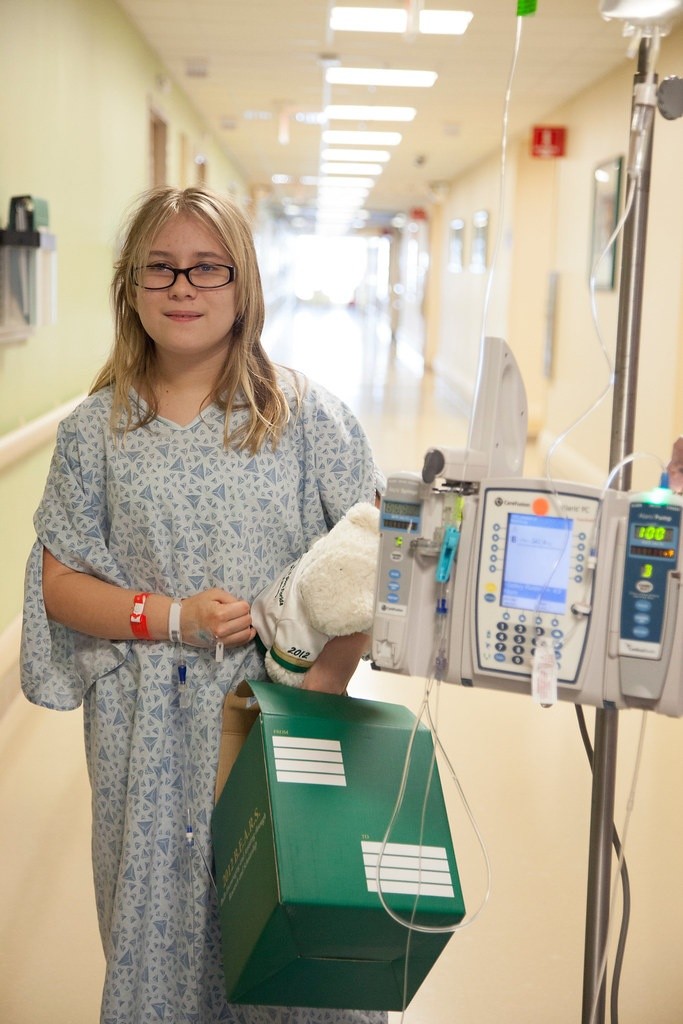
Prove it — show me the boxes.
[130,592,149,642]
[169,593,184,644]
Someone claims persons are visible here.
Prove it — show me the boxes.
[17,188,392,1024]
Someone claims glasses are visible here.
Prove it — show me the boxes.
[131,259,235,291]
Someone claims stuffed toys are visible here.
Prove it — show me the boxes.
[251,501,380,688]
